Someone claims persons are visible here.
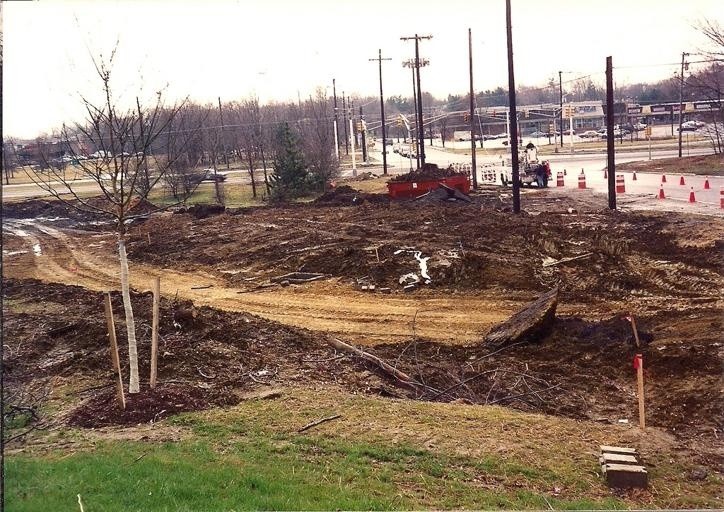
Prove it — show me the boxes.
[523,142,539,164]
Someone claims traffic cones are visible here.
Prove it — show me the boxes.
[658,184,665,200]
[632,170,637,181]
[704,174,710,190]
[582,168,584,174]
[689,186,696,204]
[563,168,567,176]
[679,176,685,185]
[604,169,607,178]
[661,174,666,182]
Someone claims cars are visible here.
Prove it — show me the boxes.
[184,169,228,183]
[502,139,523,146]
[578,122,648,138]
[676,119,707,131]
[472,132,509,141]
[393,137,426,160]
[384,138,393,146]
[530,128,576,139]
[46,150,145,163]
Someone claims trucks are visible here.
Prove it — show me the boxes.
[500,156,549,190]
